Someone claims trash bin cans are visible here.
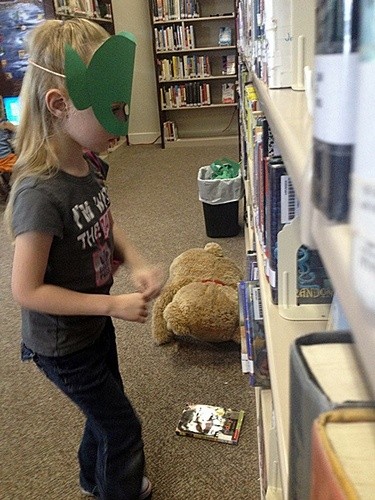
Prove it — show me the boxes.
[197,165,244,240]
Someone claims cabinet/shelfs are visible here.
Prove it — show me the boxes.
[149,0,240,149]
[239,1,375,500]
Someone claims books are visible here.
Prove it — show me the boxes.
[237,56,332,306]
[222,55,235,75]
[287,332,374,500]
[160,82,210,108]
[237,250,271,389]
[54,0,111,18]
[236,0,293,89]
[222,83,234,104]
[155,25,195,52]
[163,121,176,142]
[313,0,374,316]
[175,404,245,445]
[157,55,211,81]
[149,0,200,21]
[218,27,231,46]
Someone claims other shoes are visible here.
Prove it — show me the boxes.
[80,476,152,498]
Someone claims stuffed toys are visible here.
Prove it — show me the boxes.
[152,242,248,345]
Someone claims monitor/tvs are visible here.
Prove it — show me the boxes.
[2,96,21,127]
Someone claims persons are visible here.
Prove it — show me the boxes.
[4,20,164,500]
[0,105,18,172]
